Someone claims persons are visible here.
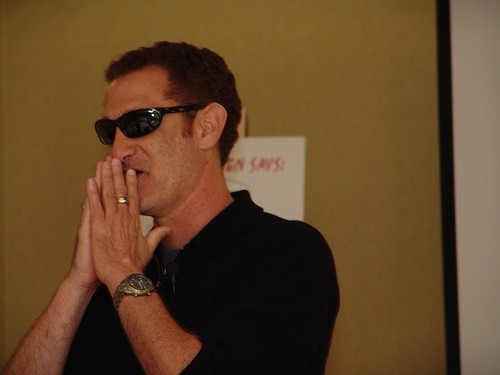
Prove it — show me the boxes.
[0,41,340,375]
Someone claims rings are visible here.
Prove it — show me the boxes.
[116,197,130,205]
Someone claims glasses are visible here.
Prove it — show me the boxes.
[94,103,206,145]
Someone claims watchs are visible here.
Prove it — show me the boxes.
[113,273,157,310]
